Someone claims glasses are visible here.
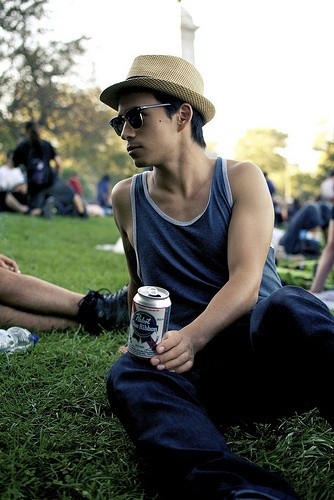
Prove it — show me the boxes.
[109,104,171,137]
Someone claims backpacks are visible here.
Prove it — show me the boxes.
[28,140,48,184]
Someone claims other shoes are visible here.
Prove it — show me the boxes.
[78,282,129,336]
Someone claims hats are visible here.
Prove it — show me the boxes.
[100,55,215,126]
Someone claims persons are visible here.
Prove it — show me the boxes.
[0,254,129,336]
[262,165,334,293]
[99,55,334,500]
[0,121,115,218]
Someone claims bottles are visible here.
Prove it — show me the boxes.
[0,327,39,353]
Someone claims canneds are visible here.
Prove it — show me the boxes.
[127,286,171,359]
